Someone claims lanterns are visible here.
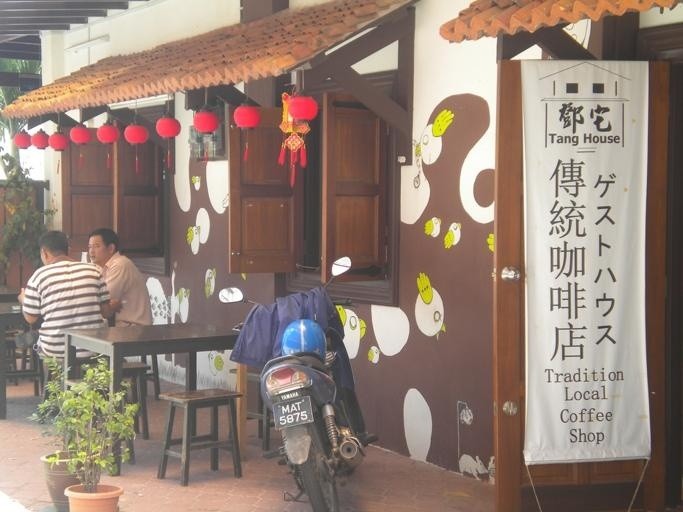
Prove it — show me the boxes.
[193,104,218,165]
[156,113,181,169]
[13,122,149,174]
[234,99,260,160]
[288,88,318,145]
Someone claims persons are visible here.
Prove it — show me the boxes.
[88,228,153,327]
[18,231,122,425]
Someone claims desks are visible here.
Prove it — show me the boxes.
[62,323,247,476]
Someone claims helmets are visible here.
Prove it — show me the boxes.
[282,320,326,363]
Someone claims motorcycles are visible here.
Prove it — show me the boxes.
[216,253,380,512]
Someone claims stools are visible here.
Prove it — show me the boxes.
[120,354,162,398]
[230,369,276,449]
[0,285,96,419]
[89,362,150,439]
[158,386,242,486]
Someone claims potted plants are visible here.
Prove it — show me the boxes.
[59,366,140,512]
[25,355,101,512]
